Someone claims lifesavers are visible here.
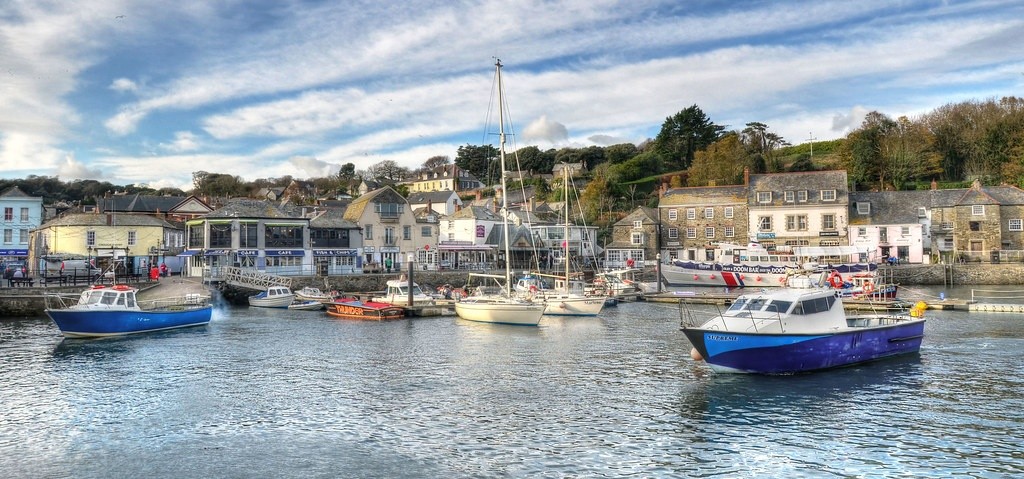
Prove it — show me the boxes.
[862,282,875,293]
[91,285,105,289]
[112,285,128,290]
[830,272,843,288]
[530,285,537,292]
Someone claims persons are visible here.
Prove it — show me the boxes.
[386,257,392,274]
[538,254,553,270]
[160,262,167,278]
[888,256,897,266]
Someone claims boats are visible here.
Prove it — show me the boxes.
[657,217,899,298]
[372,279,431,306]
[288,285,340,311]
[322,294,404,321]
[585,268,641,292]
[679,286,926,376]
[248,286,295,308]
[44,285,214,340]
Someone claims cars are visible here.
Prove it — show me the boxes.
[3,264,30,279]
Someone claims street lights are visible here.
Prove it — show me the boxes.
[125,246,130,283]
[43,244,50,287]
[88,246,93,285]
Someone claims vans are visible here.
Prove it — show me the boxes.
[60,260,102,281]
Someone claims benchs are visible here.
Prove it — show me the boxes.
[885,258,901,266]
[9,273,142,287]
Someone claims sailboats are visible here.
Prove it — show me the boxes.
[454,56,549,327]
[531,161,608,317]
